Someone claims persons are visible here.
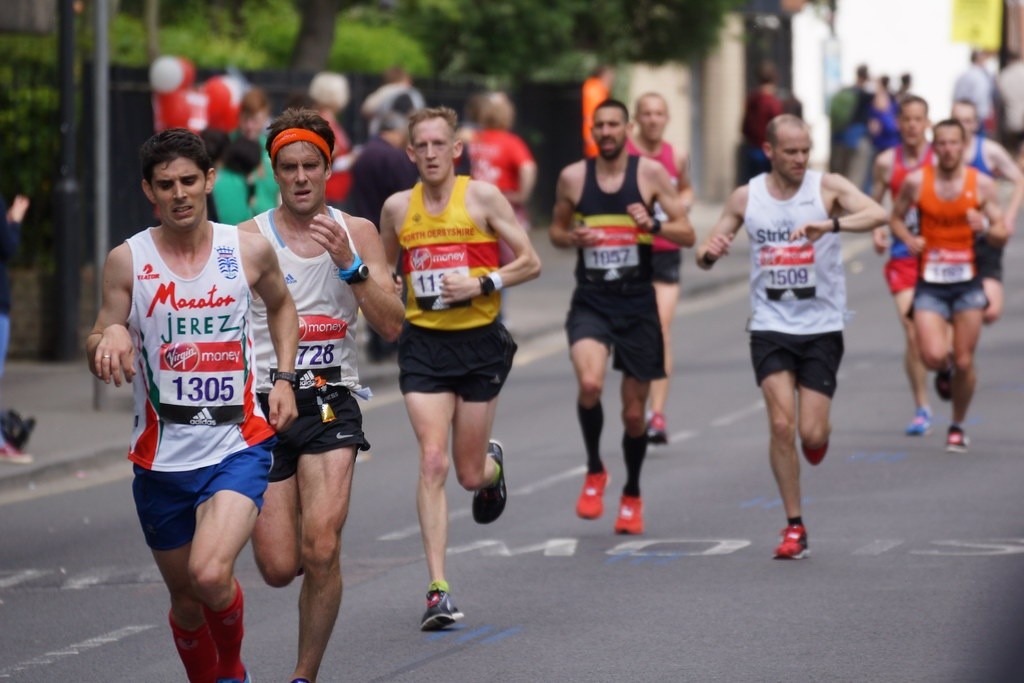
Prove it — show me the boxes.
[381,106,542,629]
[86,63,298,683]
[831,52,1024,452]
[236,107,407,683]
[695,114,892,559]
[736,59,802,185]
[198,72,536,364]
[0,186,32,463]
[550,64,695,534]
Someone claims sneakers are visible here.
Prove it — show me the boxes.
[421,588,464,629]
[473,439,507,524]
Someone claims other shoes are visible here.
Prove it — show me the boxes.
[802,442,828,465]
[775,527,810,559]
[945,426,970,453]
[936,353,957,400]
[576,470,607,518]
[614,496,642,534]
[906,409,936,435]
[646,414,668,444]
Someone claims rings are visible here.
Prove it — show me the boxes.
[103,355,111,358]
[635,215,640,220]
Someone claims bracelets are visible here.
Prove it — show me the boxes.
[339,256,362,281]
[976,217,989,238]
[647,219,664,234]
[832,215,840,234]
[489,270,503,291]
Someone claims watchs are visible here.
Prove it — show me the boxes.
[273,369,300,389]
[347,264,370,284]
[479,275,494,297]
